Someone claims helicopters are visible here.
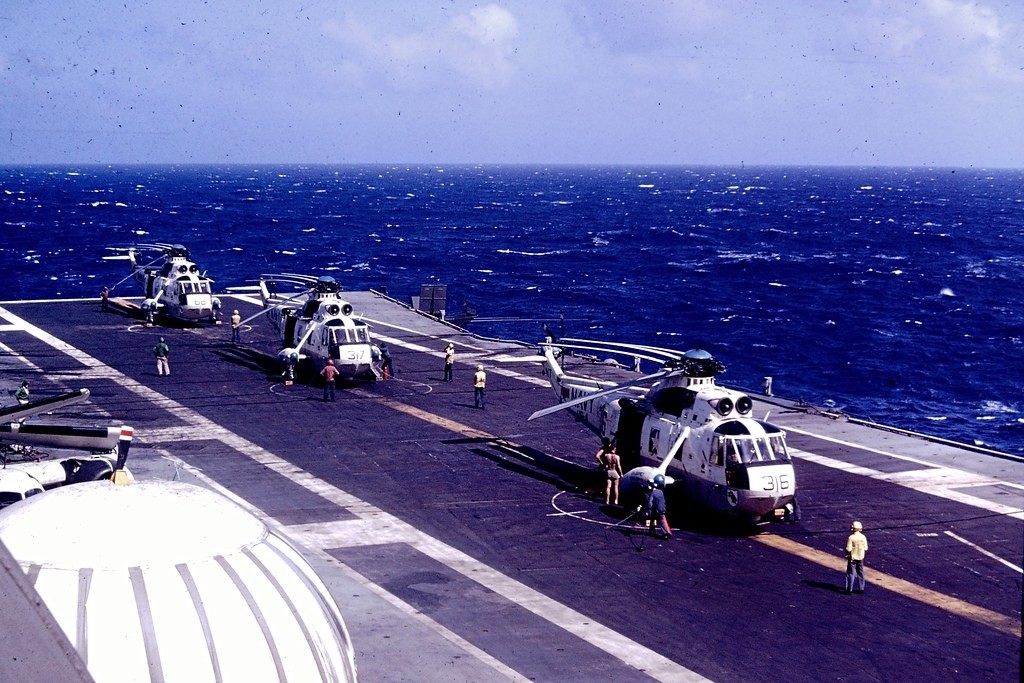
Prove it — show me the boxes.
[495,336,799,534]
[99,240,222,324]
[231,272,379,387]
[1,388,136,511]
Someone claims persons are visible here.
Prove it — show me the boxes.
[845,520,868,595]
[380,343,394,378]
[596,437,611,494]
[473,365,486,410]
[100,286,109,312]
[604,445,623,505]
[320,360,339,402]
[153,337,171,377]
[231,310,242,342]
[16,379,30,405]
[445,343,454,382]
[643,479,672,539]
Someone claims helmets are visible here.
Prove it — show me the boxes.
[233,309,239,314]
[159,337,165,343]
[327,359,334,366]
[476,364,483,371]
[448,342,454,348]
[850,521,862,531]
